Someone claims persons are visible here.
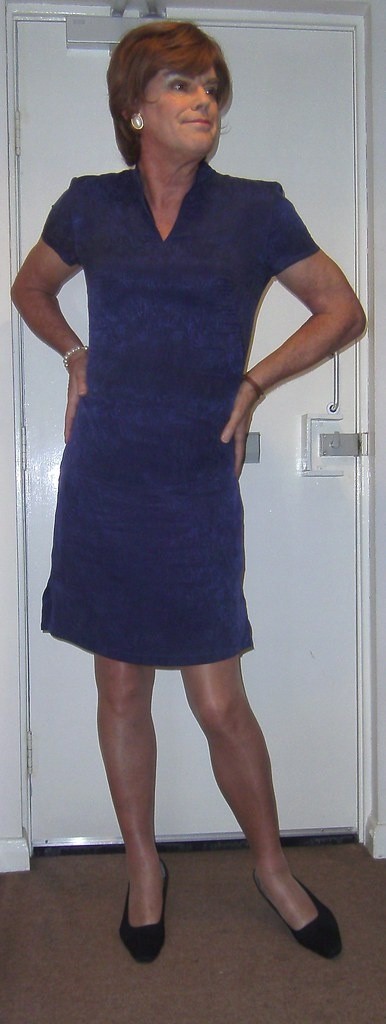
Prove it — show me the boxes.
[9,16,369,962]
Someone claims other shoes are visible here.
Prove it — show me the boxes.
[252,868,341,960]
[119,858,169,964]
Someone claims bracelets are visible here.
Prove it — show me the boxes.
[59,345,93,371]
[240,370,266,399]
